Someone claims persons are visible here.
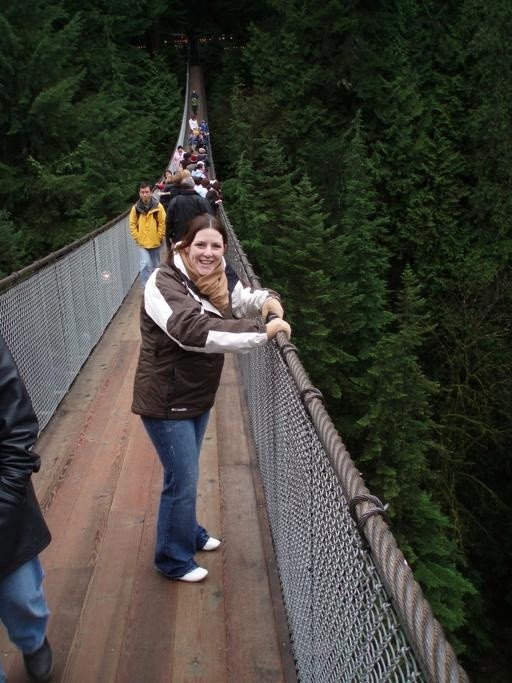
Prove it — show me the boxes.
[130,88,222,289]
[135,211,291,580]
[0,334,56,677]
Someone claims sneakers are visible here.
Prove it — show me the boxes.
[174,565,209,582]
[202,535,222,552]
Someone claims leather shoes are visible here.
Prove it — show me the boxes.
[23,635,54,682]
